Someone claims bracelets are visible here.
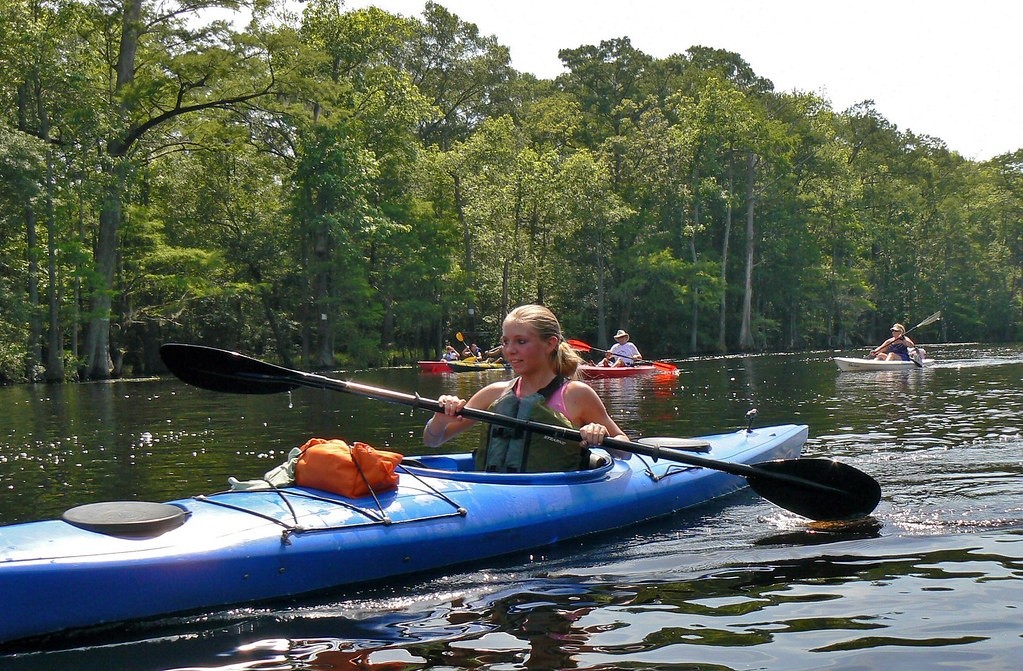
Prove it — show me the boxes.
[427,421,442,436]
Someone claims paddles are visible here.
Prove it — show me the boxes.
[875,310,942,353]
[159,341,882,522]
[456,332,480,363]
[567,337,677,372]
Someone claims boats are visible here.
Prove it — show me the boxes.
[446,360,509,372]
[834,357,935,372]
[417,360,449,372]
[554,365,656,379]
[0,424,812,643]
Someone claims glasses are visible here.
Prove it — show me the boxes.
[891,329,899,332]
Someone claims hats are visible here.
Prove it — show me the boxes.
[613,330,629,342]
[890,323,905,333]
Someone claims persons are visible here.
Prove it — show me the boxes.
[440,346,460,362]
[870,324,914,361]
[422,305,629,474]
[462,344,482,362]
[485,336,505,364]
[603,330,642,368]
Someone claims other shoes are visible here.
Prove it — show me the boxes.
[603,358,608,367]
[589,360,595,366]
